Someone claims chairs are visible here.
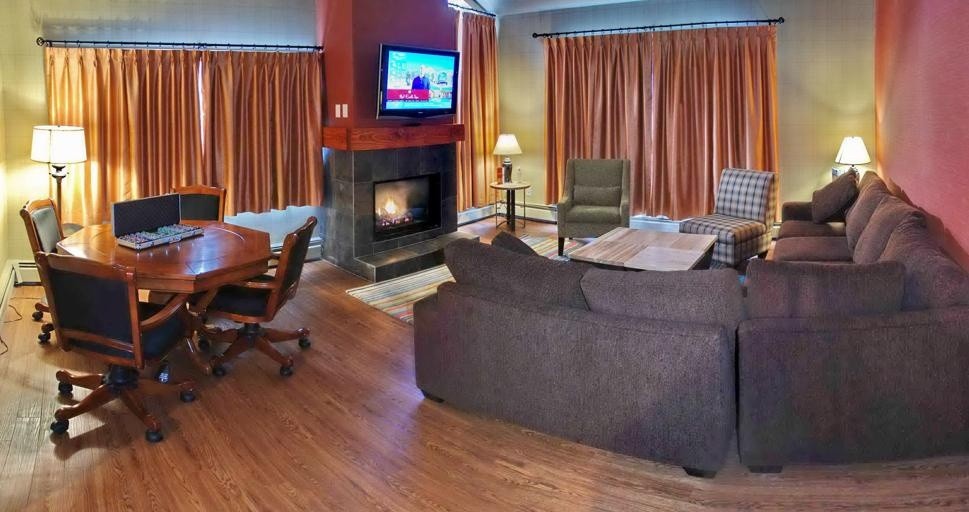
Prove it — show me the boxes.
[198,216,317,377]
[35,251,195,444]
[679,167,775,267]
[170,185,227,223]
[20,198,72,341]
[557,158,633,256]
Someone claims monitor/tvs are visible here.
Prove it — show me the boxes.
[376,44,461,127]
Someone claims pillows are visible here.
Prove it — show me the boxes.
[845,181,889,256]
[852,195,924,262]
[812,169,858,223]
[445,238,591,308]
[881,217,969,311]
[746,259,906,317]
[581,267,742,329]
[843,171,880,219]
[492,230,537,255]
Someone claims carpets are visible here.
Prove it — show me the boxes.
[346,230,588,328]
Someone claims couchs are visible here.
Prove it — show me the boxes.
[415,238,745,477]
[737,169,969,474]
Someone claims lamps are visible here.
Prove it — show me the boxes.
[30,126,87,219]
[493,134,522,184]
[835,136,871,169]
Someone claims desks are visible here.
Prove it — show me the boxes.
[490,182,530,228]
[57,218,272,376]
